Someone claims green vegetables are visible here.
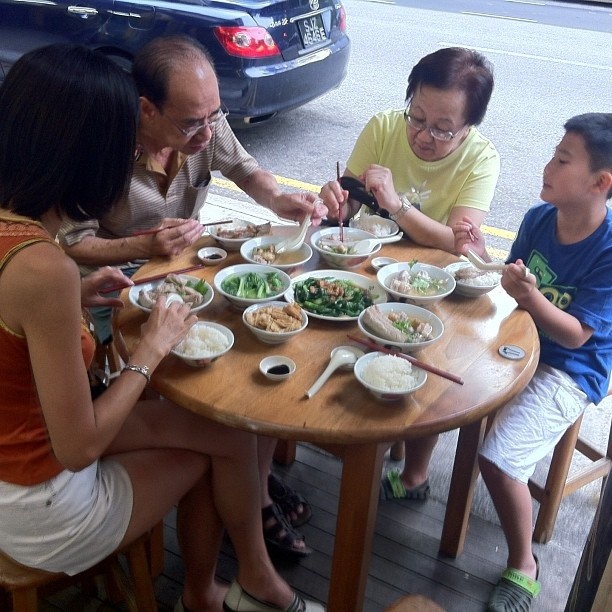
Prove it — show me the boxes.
[222,270,280,301]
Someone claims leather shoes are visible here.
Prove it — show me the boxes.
[173,594,185,612]
[223,578,325,612]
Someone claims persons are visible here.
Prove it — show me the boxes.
[452,112,612,609]
[1,44,314,604]
[57,34,330,560]
[319,45,501,509]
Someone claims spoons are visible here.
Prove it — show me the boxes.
[305,349,357,398]
[275,199,323,252]
[349,232,403,253]
[467,249,530,278]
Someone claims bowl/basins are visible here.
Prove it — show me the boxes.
[169,321,235,369]
[377,261,457,308]
[353,351,427,404]
[207,219,261,251]
[129,274,215,320]
[349,217,400,238]
[240,236,313,275]
[357,302,445,354]
[445,261,502,299]
[310,227,382,270]
[241,301,309,345]
[213,264,291,312]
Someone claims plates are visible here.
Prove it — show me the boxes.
[283,269,387,321]
[198,246,227,265]
[371,257,399,270]
[331,345,365,371]
[258,356,296,381]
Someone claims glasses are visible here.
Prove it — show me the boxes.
[126,141,145,161]
[165,99,229,137]
[404,96,471,141]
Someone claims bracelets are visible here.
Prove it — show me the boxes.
[118,364,149,379]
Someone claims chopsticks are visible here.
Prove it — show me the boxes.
[97,265,206,294]
[346,334,464,386]
[337,162,344,242]
[133,220,233,237]
[466,227,479,242]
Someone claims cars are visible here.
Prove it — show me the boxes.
[0,0,349,129]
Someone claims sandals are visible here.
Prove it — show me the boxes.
[261,502,313,557]
[377,467,430,501]
[268,473,313,528]
[487,552,541,612]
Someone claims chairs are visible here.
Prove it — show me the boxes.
[0,538,158,612]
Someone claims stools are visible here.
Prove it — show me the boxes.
[510,376,612,546]
[113,225,541,612]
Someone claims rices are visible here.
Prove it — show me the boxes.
[217,222,256,240]
[357,216,394,241]
[360,352,417,390]
[455,264,497,285]
[178,325,231,356]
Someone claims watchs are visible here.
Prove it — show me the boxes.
[389,196,412,222]
